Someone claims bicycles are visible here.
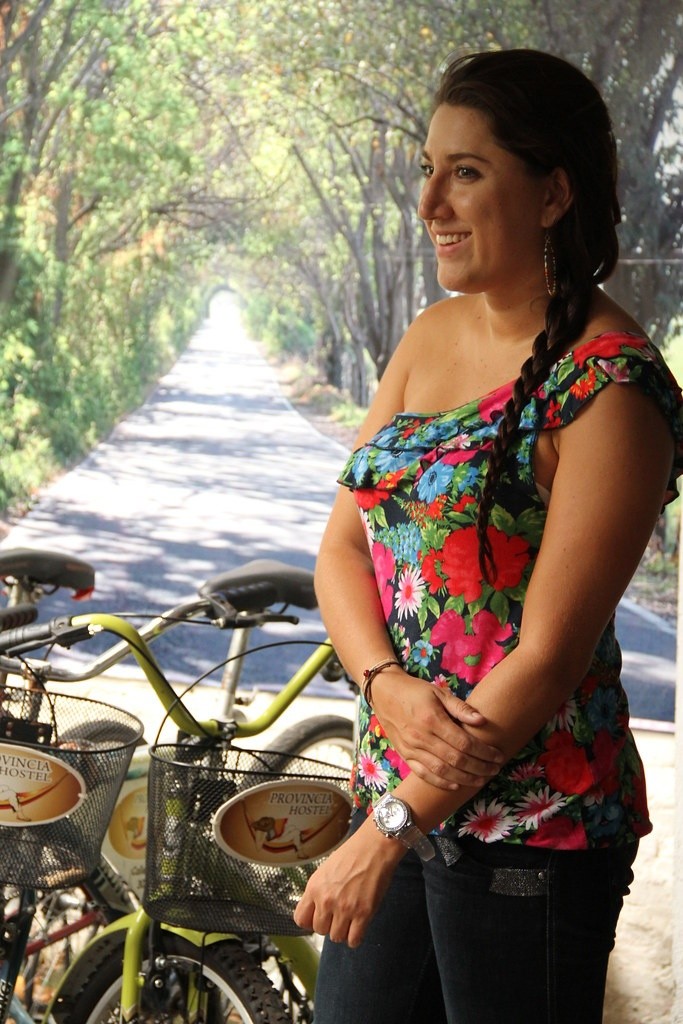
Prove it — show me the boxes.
[0,545,369,1024]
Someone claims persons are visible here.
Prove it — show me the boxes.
[292,49,683,1024]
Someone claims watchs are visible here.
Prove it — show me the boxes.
[372,791,436,861]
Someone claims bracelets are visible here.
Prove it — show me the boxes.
[361,659,401,709]
